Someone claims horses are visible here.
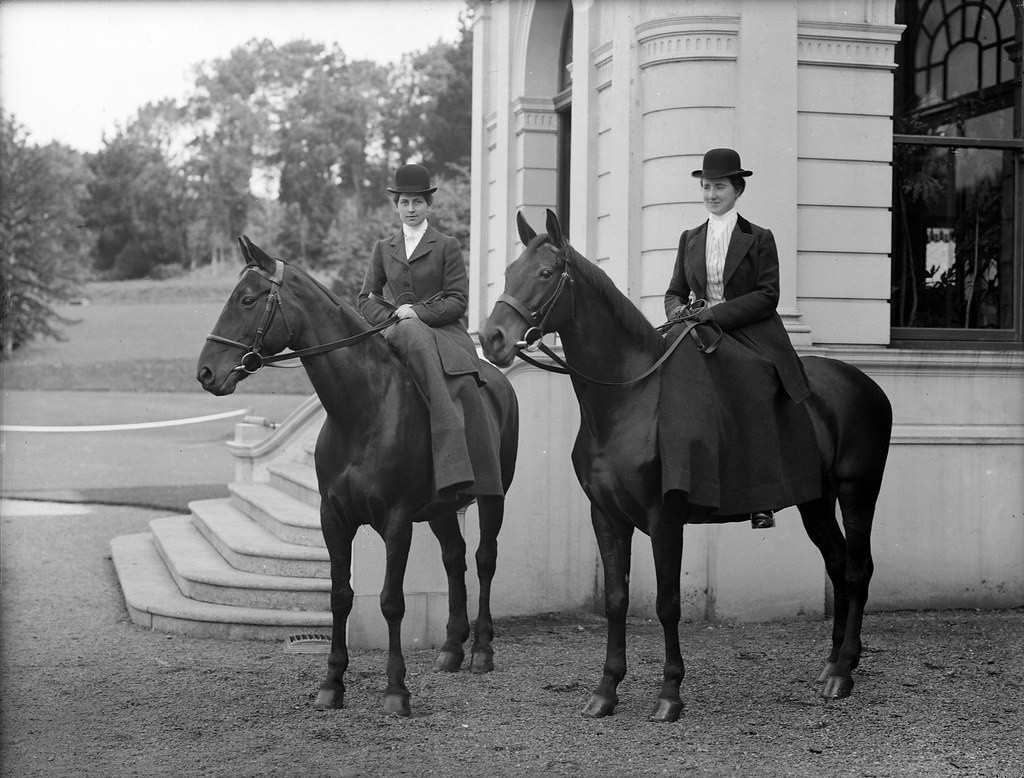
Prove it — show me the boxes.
[196,234,519,712]
[478,207,892,723]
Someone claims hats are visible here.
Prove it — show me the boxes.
[387,164,437,194]
[692,149,752,179]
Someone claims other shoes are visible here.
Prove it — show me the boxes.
[750,511,774,528]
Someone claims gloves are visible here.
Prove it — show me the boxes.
[395,307,416,318]
[693,307,712,321]
[670,307,689,320]
[392,304,413,317]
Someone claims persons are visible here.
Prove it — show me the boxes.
[356,164,505,505]
[660,145,818,529]
